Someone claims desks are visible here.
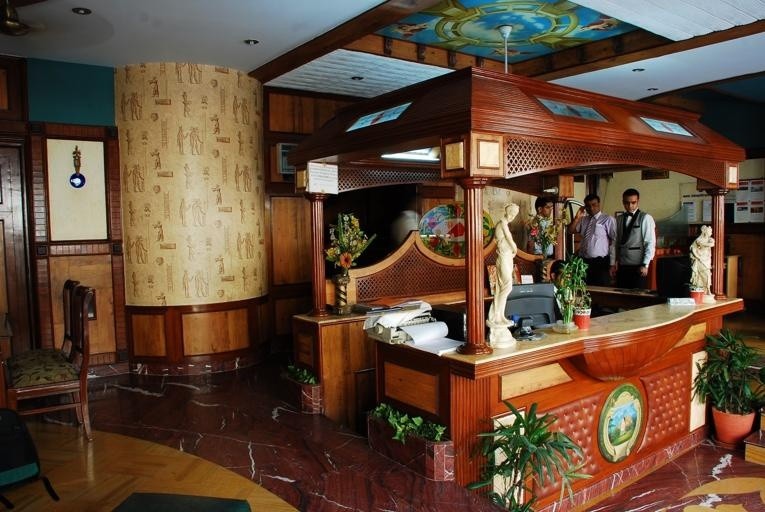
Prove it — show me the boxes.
[647,247,695,292]
[0,311,15,405]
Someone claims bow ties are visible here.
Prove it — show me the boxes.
[625,211,634,216]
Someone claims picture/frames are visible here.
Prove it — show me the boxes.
[42,135,113,243]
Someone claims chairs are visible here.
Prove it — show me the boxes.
[0,275,100,445]
[0,406,63,511]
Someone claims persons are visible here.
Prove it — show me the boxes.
[528,196,586,280]
[546,258,584,315]
[490,201,520,324]
[690,226,717,290]
[567,192,617,287]
[609,188,657,291]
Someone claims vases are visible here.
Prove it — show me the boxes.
[329,273,354,316]
[533,241,554,255]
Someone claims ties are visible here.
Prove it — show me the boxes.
[581,216,594,257]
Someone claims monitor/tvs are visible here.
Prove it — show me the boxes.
[504,283,556,341]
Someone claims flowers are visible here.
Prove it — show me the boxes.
[524,206,568,256]
[320,211,379,278]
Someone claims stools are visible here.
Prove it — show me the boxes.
[106,486,257,510]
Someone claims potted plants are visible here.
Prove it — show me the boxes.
[557,255,594,332]
[551,286,580,336]
[283,363,323,415]
[687,280,707,306]
[463,396,599,511]
[686,323,764,452]
[365,398,459,484]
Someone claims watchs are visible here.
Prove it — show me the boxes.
[642,263,650,268]
